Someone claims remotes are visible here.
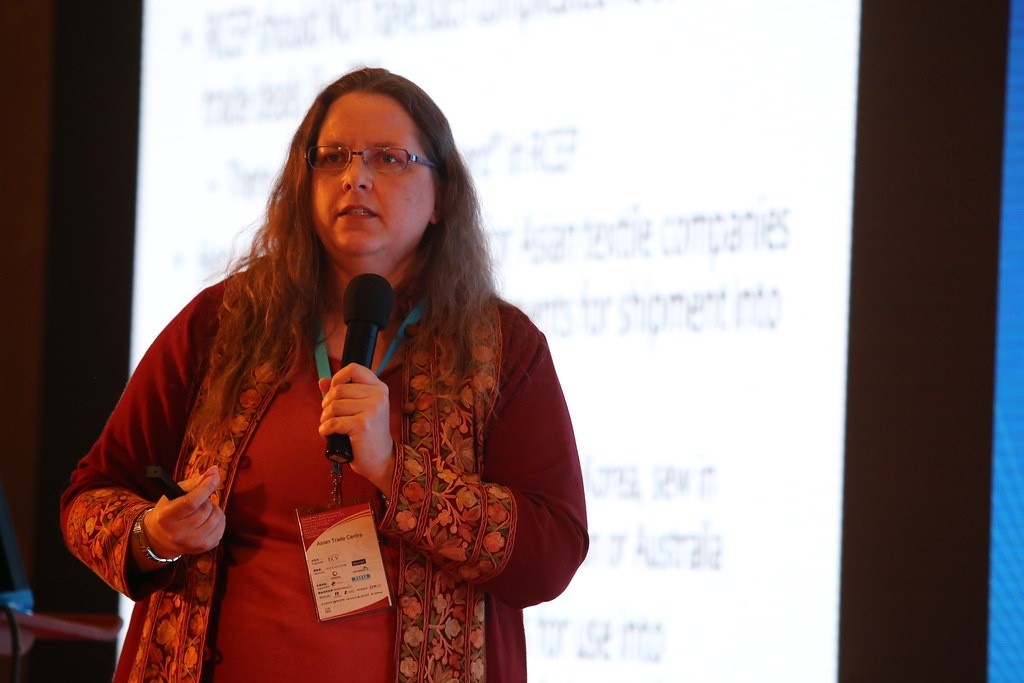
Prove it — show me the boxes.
[145,466,187,501]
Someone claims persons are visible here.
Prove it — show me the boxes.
[55,69,588,682]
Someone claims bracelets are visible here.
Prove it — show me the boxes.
[134,507,183,564]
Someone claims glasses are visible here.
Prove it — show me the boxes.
[305,145,445,174]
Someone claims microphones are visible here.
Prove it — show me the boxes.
[325,274,394,462]
